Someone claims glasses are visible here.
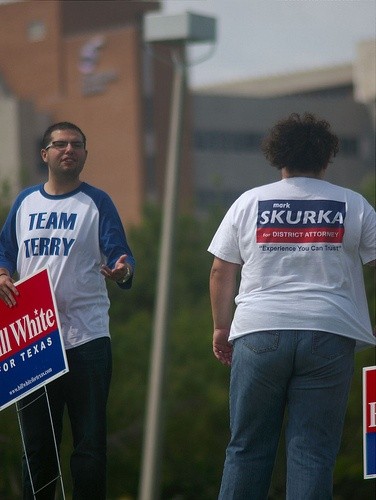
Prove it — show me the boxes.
[44,140,85,151]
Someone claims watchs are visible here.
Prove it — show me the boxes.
[117,267,130,284]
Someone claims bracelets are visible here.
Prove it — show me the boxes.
[0,273,9,277]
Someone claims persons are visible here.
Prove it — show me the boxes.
[205,112,376,500]
[1,120,138,499]
